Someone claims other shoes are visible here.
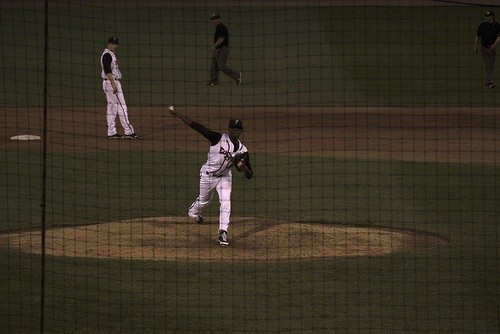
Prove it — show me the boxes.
[237,72,241,85]
[217,234,229,245]
[194,217,203,223]
[108,135,123,139]
[125,134,141,139]
[208,83,219,86]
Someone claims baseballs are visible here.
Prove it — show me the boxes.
[169,105,175,111]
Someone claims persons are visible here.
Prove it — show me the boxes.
[474,11,500,89]
[170,108,252,245]
[100,37,143,138]
[206,13,243,86]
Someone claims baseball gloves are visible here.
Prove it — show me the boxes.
[236,153,247,171]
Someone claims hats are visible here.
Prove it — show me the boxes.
[228,119,243,128]
[210,13,219,19]
[108,37,120,44]
